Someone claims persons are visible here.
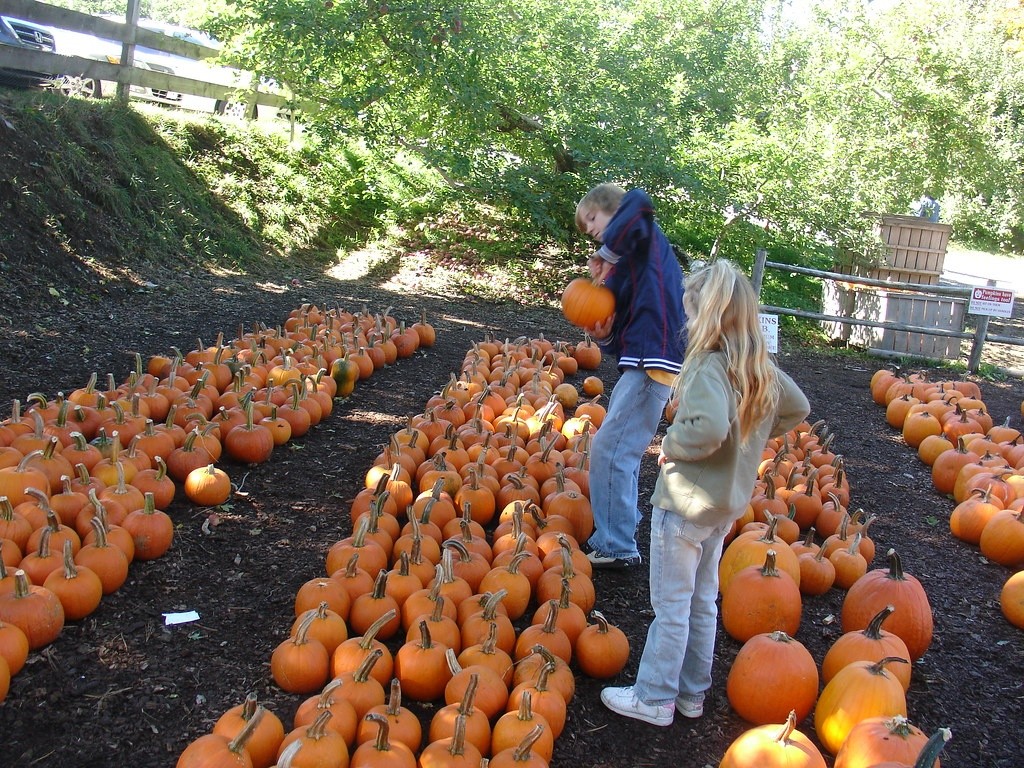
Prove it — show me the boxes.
[574,182,689,568]
[599,256,812,727]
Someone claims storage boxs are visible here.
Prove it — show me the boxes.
[834,207,955,285]
[819,278,856,342]
[849,287,969,359]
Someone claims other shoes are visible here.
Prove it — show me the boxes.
[579,541,641,568]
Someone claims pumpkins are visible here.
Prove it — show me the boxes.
[561,277,615,330]
[0,297,437,702]
[177,331,628,768]
[666,390,950,768]
[871,362,1024,631]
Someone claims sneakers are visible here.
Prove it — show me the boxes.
[676,696,705,718]
[600,685,675,727]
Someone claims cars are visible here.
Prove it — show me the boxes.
[48,27,185,108]
[113,15,307,120]
[0,15,57,89]
